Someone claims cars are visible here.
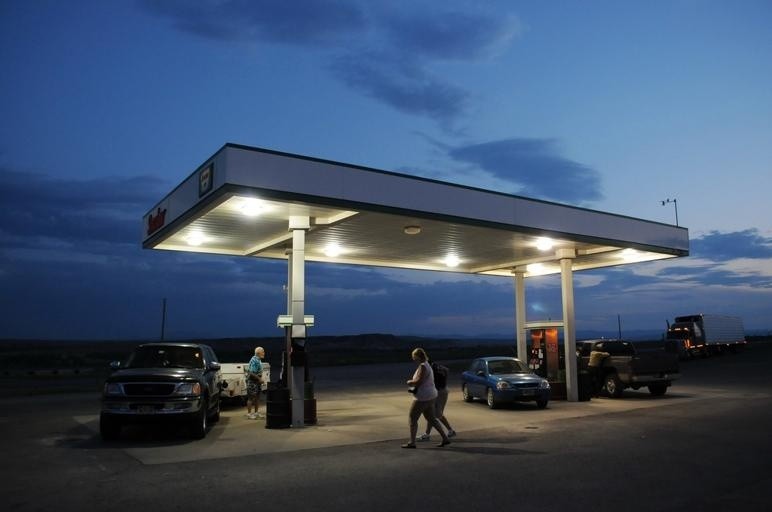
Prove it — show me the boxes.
[462,356,551,408]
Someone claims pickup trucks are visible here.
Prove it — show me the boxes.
[563,341,681,397]
[198,344,270,406]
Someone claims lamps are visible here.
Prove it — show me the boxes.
[404,226,421,235]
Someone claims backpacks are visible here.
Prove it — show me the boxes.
[433,362,449,390]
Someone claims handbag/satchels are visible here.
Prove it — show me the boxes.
[408,385,418,394]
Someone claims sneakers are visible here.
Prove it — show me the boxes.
[401,442,416,448]
[246,412,265,420]
[448,430,457,439]
[437,439,451,447]
[416,433,432,441]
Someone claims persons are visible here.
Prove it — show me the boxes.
[243,346,266,421]
[415,356,456,441]
[585,345,611,399]
[399,348,452,449]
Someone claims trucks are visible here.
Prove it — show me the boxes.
[664,314,747,360]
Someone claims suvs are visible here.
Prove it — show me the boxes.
[99,343,223,439]
